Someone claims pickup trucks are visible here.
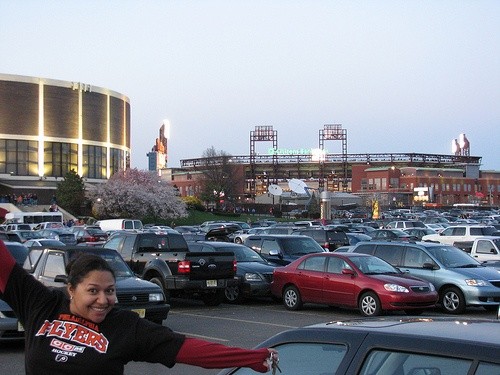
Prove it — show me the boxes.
[102,233,236,303]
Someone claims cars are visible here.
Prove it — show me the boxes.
[422,234,500,262]
[196,241,276,306]
[0,241,31,346]
[438,225,499,235]
[271,252,439,318]
[1,208,500,242]
[212,317,500,375]
[241,235,325,269]
[27,247,169,325]
[349,241,500,314]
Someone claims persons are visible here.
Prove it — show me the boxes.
[0,193,39,207]
[63,218,85,227]
[0,239,278,375]
[49,203,59,212]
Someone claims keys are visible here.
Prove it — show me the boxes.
[267,360,273,371]
